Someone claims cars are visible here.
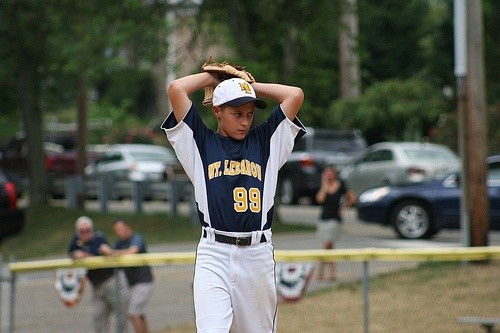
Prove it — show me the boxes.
[337,140,462,207]
[356,154,500,241]
[0,132,195,242]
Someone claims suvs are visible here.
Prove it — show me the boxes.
[275,126,371,207]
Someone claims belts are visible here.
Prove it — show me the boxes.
[201,226,267,246]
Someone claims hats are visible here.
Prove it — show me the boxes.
[212,78,267,109]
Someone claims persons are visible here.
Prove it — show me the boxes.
[160,56,307,333]
[99,218,154,333]
[315,164,357,282]
[67,216,131,333]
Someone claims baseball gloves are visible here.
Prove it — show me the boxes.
[200,61,255,107]
